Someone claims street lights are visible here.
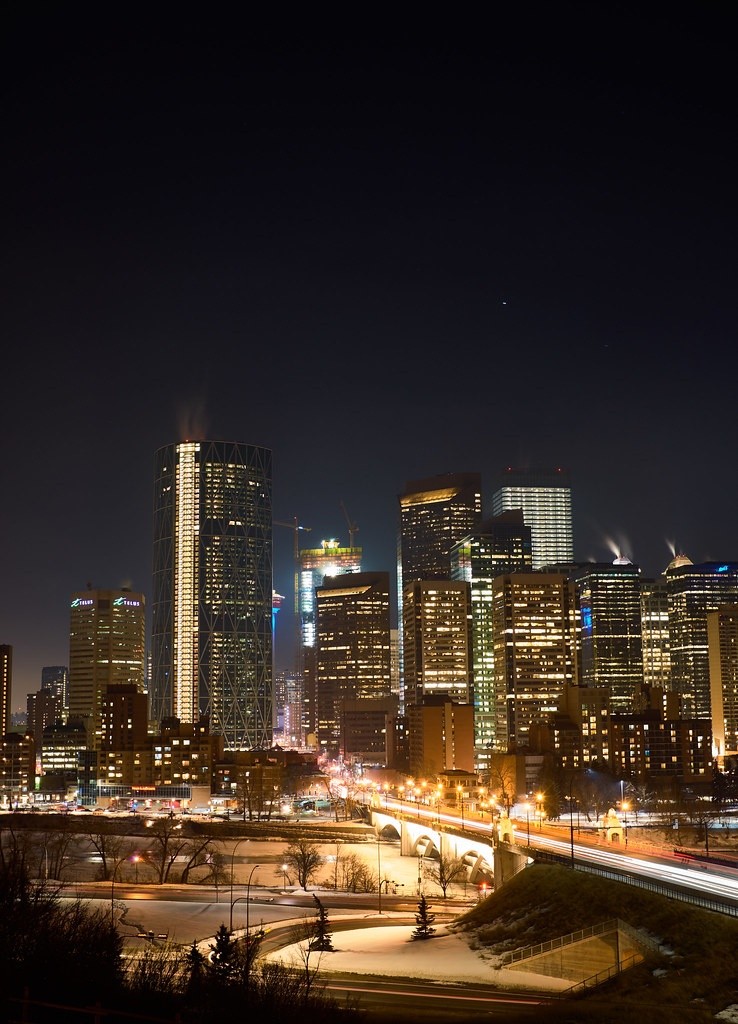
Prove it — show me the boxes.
[283,865,287,892]
[538,795,542,827]
[525,804,530,847]
[458,786,462,809]
[416,790,420,818]
[111,858,125,925]
[135,857,139,883]
[623,804,628,844]
[230,838,254,935]
[399,787,404,813]
[435,792,441,823]
[480,789,484,818]
[570,769,590,869]
[384,785,388,810]
[247,866,259,950]
[378,828,390,914]
[490,799,494,823]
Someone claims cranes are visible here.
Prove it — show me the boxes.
[272,515,305,677]
[339,499,360,547]
